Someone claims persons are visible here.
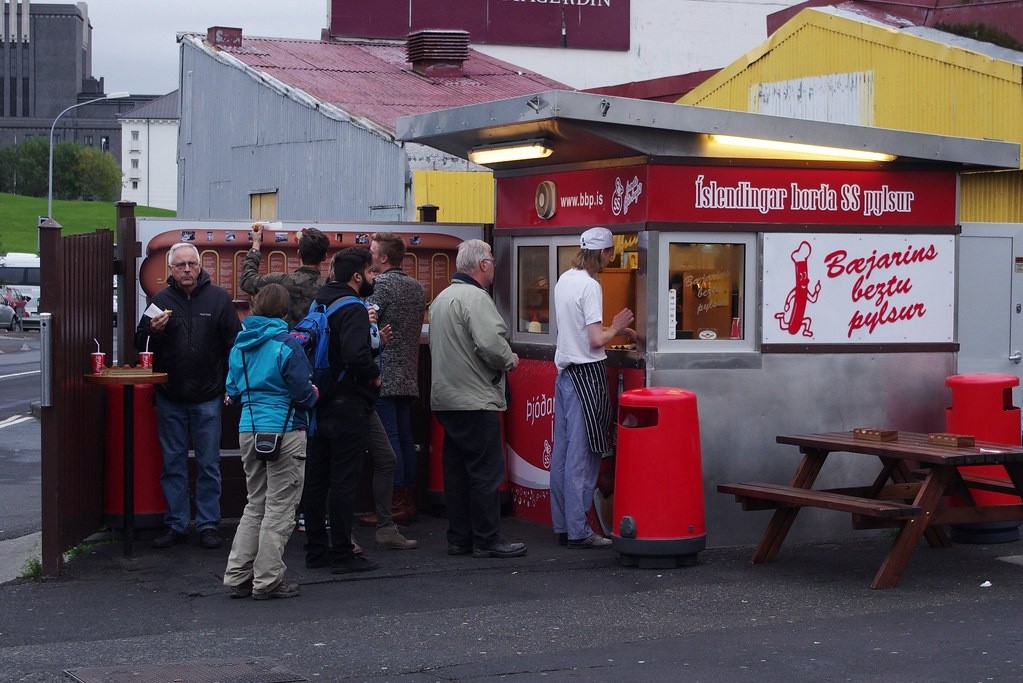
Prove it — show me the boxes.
[549,228,636,548]
[428,239,528,557]
[302,247,382,574]
[224,284,317,599]
[240,224,426,554]
[136,243,245,547]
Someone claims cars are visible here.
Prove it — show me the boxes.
[0,284,30,331]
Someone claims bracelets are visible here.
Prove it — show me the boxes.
[610,327,619,336]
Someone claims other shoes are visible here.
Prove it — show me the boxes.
[565,533,612,549]
[557,533,567,546]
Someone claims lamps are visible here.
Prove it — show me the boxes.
[468,137,557,164]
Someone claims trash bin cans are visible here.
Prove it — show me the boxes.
[103,383,169,542]
[611,386,707,567]
[944,373,1023,543]
[428,410,508,519]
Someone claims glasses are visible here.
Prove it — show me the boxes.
[173,262,200,270]
[482,259,498,267]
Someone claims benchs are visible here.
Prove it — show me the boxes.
[906,469,1023,498]
[717,482,922,519]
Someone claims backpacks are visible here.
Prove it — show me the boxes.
[289,297,371,437]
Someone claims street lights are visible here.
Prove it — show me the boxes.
[48,90,132,220]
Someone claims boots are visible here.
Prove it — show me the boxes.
[359,483,417,527]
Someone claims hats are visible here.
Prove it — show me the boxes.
[580,227,613,249]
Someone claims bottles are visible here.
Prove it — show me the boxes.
[365,301,380,349]
[528,313,541,333]
[611,374,625,448]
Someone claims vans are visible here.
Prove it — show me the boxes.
[0,252,41,317]
[112,274,118,327]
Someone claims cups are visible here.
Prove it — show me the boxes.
[91,353,106,374]
[253,225,263,241]
[730,318,741,339]
[139,351,154,367]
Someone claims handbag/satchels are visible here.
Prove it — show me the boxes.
[255,433,283,461]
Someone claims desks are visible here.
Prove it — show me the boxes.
[86,367,167,559]
[750,435,1023,590]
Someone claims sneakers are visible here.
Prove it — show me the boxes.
[351,534,363,555]
[448,543,473,556]
[229,576,255,597]
[201,529,222,548]
[472,541,526,558]
[306,554,334,568]
[375,522,417,551]
[252,580,301,600]
[152,528,188,546]
[332,554,379,573]
